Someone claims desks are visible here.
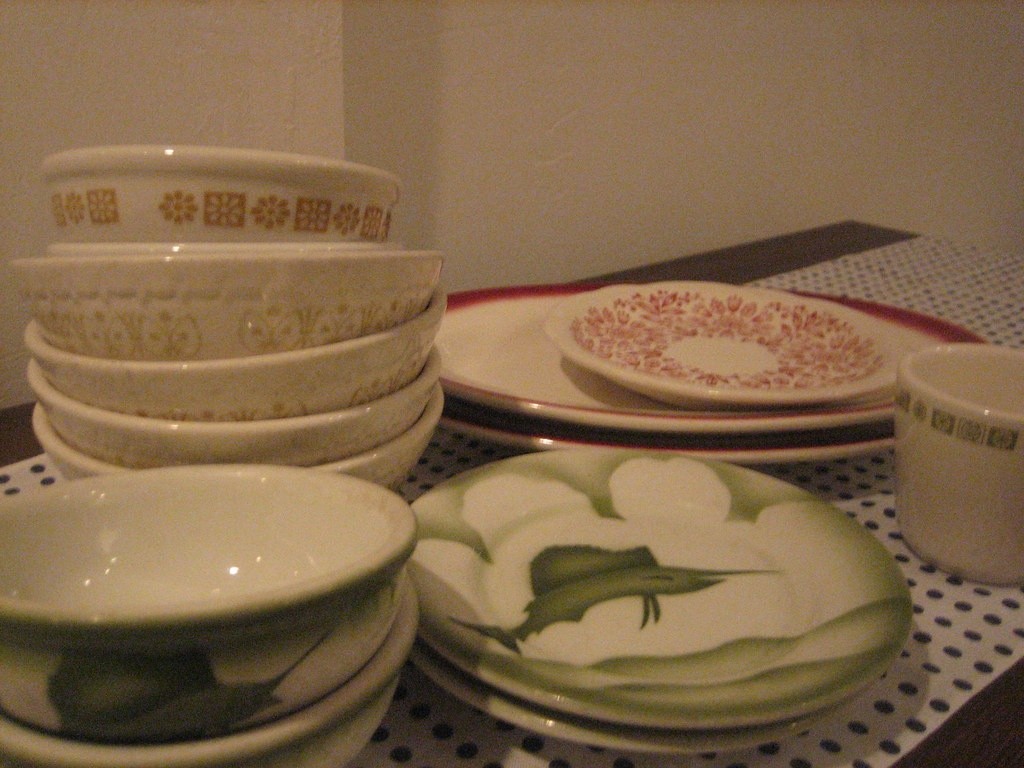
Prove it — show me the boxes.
[0,218,1024,767]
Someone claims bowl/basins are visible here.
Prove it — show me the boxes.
[9,145,445,495]
[0,462,419,768]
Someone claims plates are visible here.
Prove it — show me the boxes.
[438,281,947,458]
[404,447,914,754]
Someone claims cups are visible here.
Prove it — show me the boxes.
[895,345,1024,586]
